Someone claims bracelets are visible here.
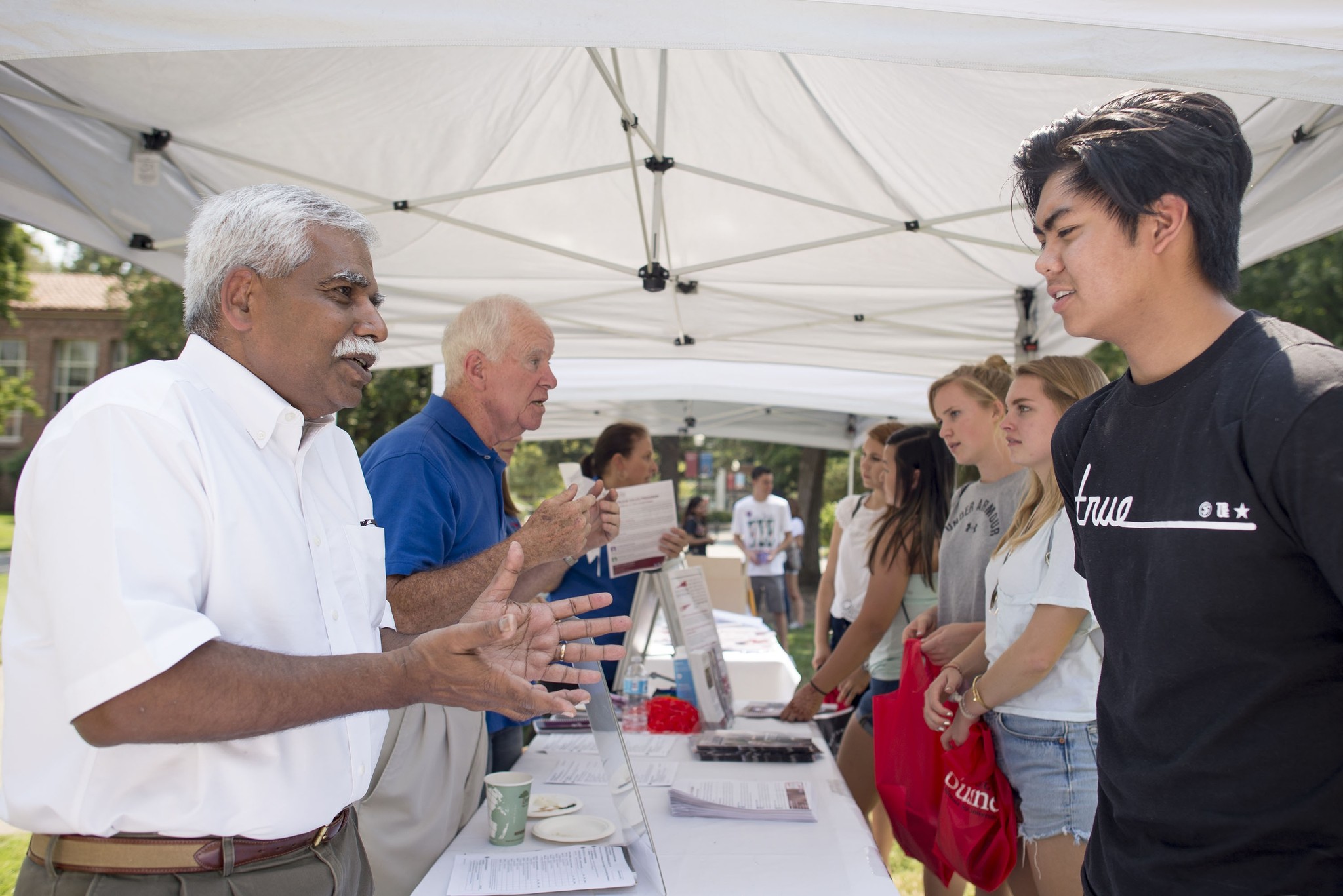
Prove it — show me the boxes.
[564,556,579,567]
[809,679,828,696]
[940,664,964,676]
[973,674,992,710]
[958,690,980,719]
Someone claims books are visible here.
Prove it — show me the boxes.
[611,564,735,730]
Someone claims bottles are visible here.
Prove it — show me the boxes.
[622,652,647,731]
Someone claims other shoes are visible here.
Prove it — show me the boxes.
[787,621,804,630]
[789,654,797,670]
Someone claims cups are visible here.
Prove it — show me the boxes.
[483,770,532,845]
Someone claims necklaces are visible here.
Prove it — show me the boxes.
[989,493,1047,613]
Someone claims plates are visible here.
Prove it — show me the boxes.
[527,793,615,845]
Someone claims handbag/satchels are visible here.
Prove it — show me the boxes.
[872,637,954,889]
[933,700,1018,893]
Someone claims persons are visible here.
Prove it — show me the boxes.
[1007,88,1343,895]
[812,423,907,867]
[900,352,1015,895]
[683,496,717,556]
[350,291,623,896]
[521,421,690,752]
[779,423,969,895]
[922,356,1112,895]
[731,466,807,654]
[0,182,635,896]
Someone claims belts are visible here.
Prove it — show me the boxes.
[27,808,350,875]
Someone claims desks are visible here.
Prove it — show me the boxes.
[410,604,901,896]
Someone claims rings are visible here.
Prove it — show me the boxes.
[559,644,566,663]
